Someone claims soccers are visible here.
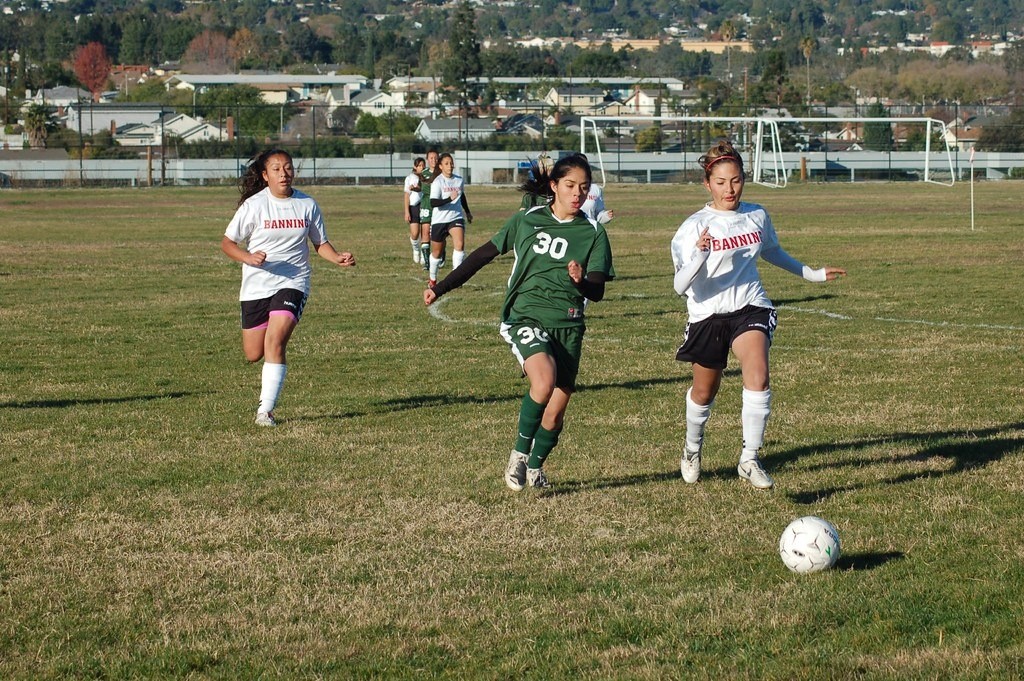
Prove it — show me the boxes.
[779,515,841,574]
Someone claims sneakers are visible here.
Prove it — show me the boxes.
[738,459,773,488]
[255,412,277,427]
[526,466,553,489]
[423,263,429,270]
[437,251,446,269]
[505,449,530,492]
[681,430,704,484]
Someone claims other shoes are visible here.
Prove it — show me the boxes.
[420,251,425,265]
[428,279,437,289]
[457,285,463,289]
[413,247,419,263]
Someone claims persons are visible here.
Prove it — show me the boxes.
[519,153,614,310]
[221,150,357,428]
[404,150,474,289]
[423,158,611,492]
[672,144,848,489]
